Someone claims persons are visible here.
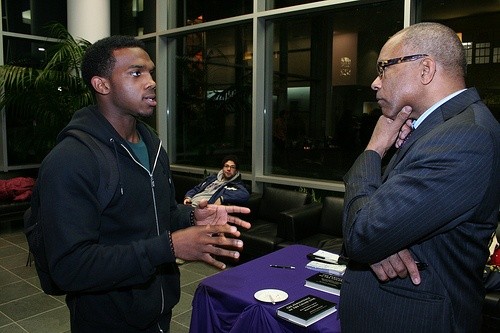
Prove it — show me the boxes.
[274,109,288,141]
[336,23,500,333]
[176,159,250,265]
[31,35,251,333]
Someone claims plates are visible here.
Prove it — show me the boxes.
[253,289,288,302]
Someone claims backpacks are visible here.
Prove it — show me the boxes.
[23,129,120,296]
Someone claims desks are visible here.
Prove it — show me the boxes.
[189,245,352,333]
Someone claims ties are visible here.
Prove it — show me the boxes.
[397,127,416,154]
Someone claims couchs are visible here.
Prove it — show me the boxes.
[217,185,345,257]
[170,175,259,233]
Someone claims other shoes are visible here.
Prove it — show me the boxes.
[176,257,186,263]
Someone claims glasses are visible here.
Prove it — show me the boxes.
[375,54,436,79]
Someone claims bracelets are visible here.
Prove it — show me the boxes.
[190,210,195,226]
[168,230,175,254]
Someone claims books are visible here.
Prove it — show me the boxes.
[305,249,346,276]
[276,295,337,328]
[305,272,343,296]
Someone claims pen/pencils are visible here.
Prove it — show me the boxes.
[416,263,428,268]
[269,264,296,269]
